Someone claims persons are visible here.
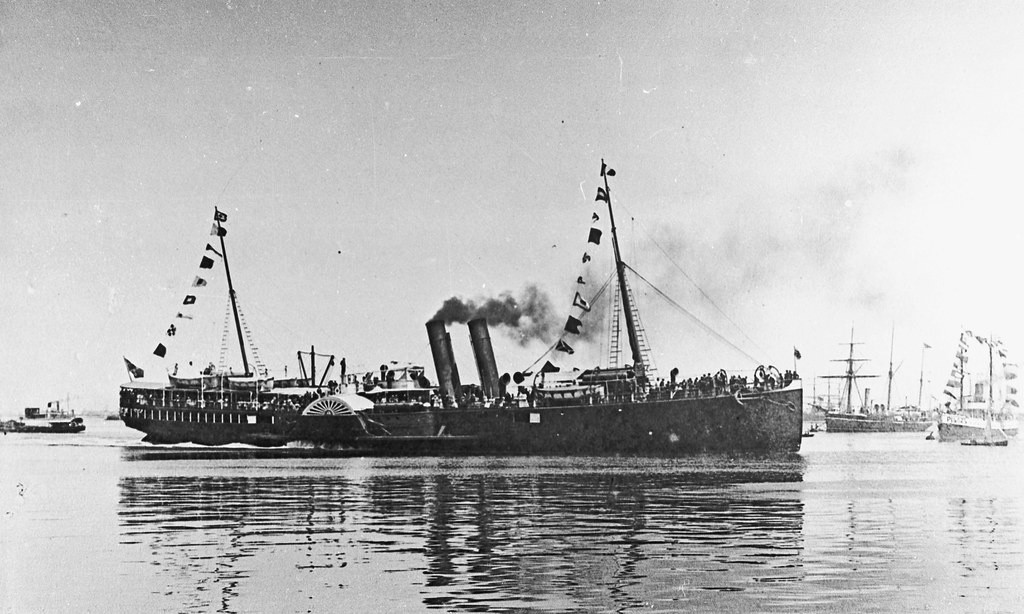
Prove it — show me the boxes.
[120,384,324,414]
[373,387,509,409]
[646,371,798,399]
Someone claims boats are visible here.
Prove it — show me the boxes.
[118,160,804,459]
[816,332,1021,447]
[0,401,85,433]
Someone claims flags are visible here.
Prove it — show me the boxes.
[193,277,207,287]
[596,187,607,203]
[541,361,560,372]
[573,292,591,311]
[601,164,616,176]
[945,329,1020,408]
[183,295,196,304]
[556,339,574,354]
[214,211,227,221]
[200,256,214,269]
[211,224,227,236]
[564,315,583,335]
[794,349,801,359]
[588,228,602,244]
[125,358,144,378]
[167,324,177,336]
[153,343,166,358]
